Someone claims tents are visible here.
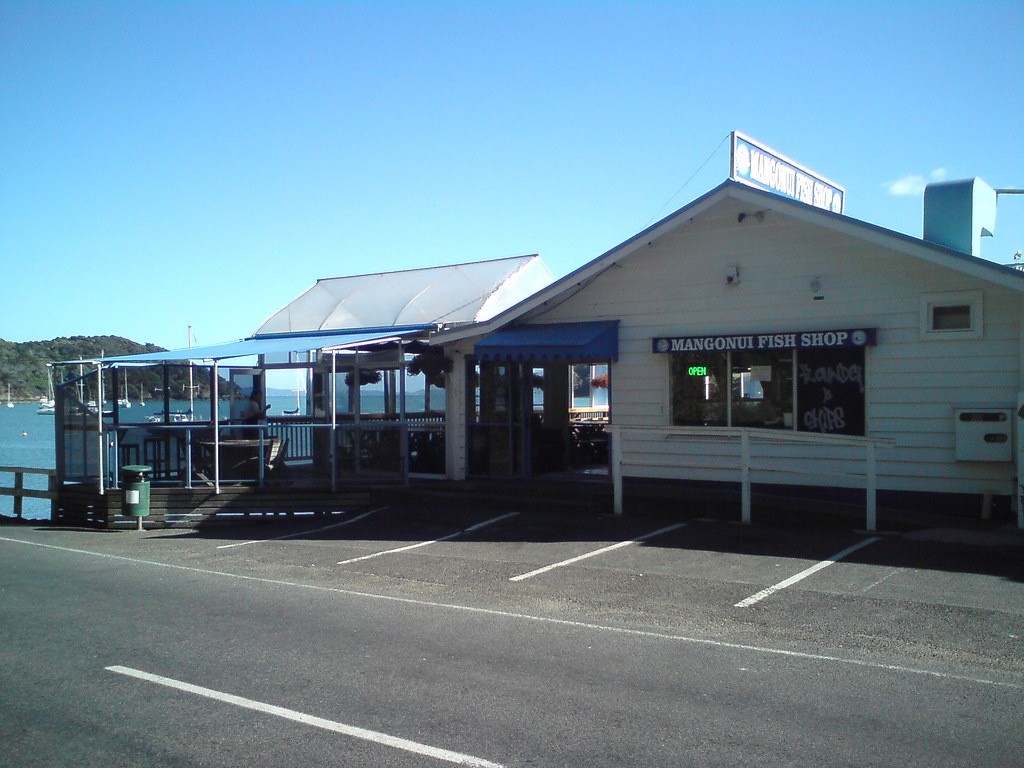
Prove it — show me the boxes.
[474,320,619,362]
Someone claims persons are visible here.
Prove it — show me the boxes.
[242,391,271,485]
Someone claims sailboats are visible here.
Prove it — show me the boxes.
[139,383,145,406]
[147,326,202,423]
[5,382,15,409]
[36,348,133,416]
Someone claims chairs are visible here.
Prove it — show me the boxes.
[247,435,290,484]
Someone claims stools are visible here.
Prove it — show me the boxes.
[142,436,166,480]
[115,443,139,489]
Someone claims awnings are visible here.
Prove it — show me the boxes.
[50,328,425,496]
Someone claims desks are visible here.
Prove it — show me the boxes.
[204,438,268,475]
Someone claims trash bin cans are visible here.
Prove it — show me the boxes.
[121,465,153,517]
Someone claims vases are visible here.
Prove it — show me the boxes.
[419,363,442,376]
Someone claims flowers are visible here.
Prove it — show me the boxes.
[406,352,454,376]
